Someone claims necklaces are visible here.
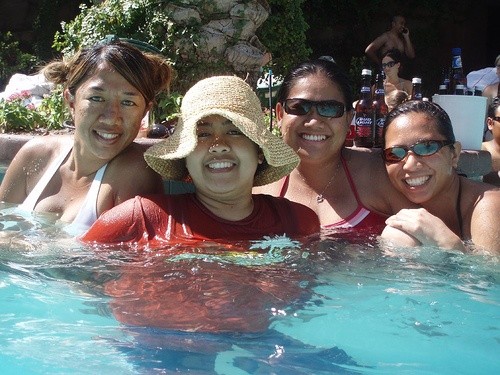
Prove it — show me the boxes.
[295,159,342,203]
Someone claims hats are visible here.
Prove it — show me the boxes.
[143,76,301,186]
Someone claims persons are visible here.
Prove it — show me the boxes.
[79,76,365,374]
[482,55,500,97]
[0,40,177,254]
[252,59,425,247]
[481,96,500,188]
[382,99,500,256]
[365,15,415,78]
[352,47,412,113]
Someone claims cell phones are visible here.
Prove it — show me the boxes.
[403,28,407,33]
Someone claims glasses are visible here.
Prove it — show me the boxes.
[282,98,352,118]
[383,139,454,164]
[491,115,500,122]
[381,61,398,69]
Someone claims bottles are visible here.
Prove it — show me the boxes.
[439,47,483,96]
[356,69,374,147]
[411,78,422,100]
[373,74,388,147]
